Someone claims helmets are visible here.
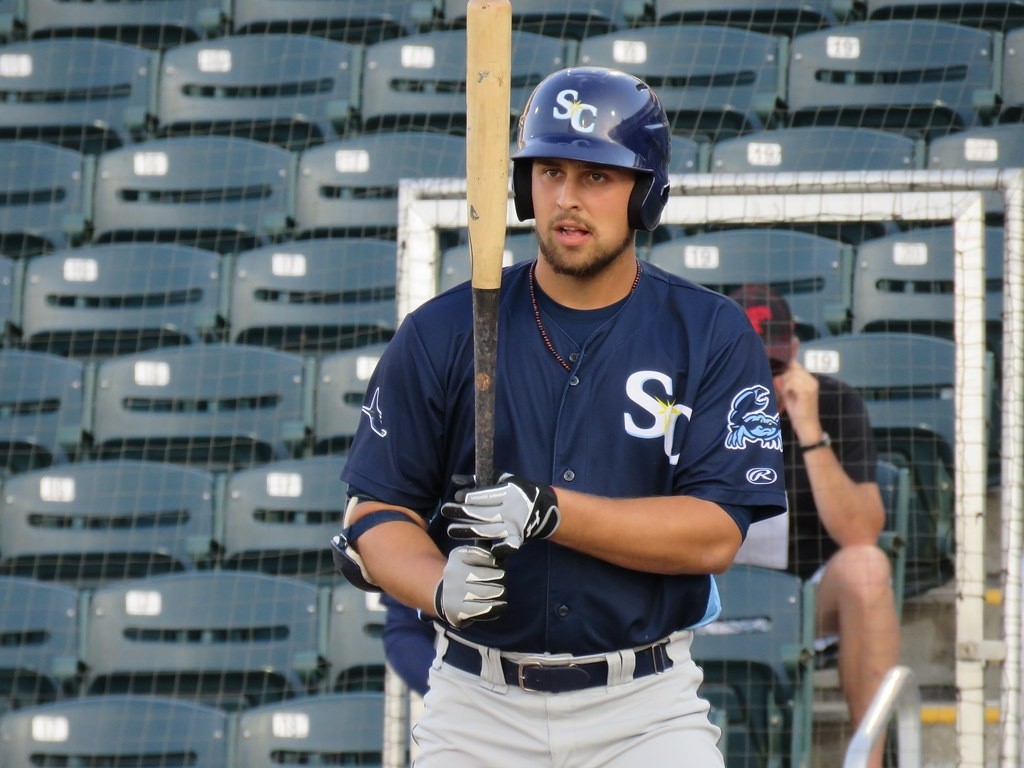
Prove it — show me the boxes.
[510,66,672,231]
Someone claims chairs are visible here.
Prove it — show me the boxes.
[2,0,1023,768]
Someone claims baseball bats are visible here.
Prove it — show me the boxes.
[459,0,516,623]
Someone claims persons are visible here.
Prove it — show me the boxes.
[329,66,787,768]
[732,285,899,767]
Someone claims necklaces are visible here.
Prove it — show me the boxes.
[528,257,641,371]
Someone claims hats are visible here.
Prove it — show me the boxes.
[729,282,795,362]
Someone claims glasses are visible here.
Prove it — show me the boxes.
[770,358,789,376]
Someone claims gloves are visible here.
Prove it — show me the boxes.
[441,471,561,558]
[433,545,508,630]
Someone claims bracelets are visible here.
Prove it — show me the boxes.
[801,434,831,451]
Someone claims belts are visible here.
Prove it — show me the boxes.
[442,633,672,694]
